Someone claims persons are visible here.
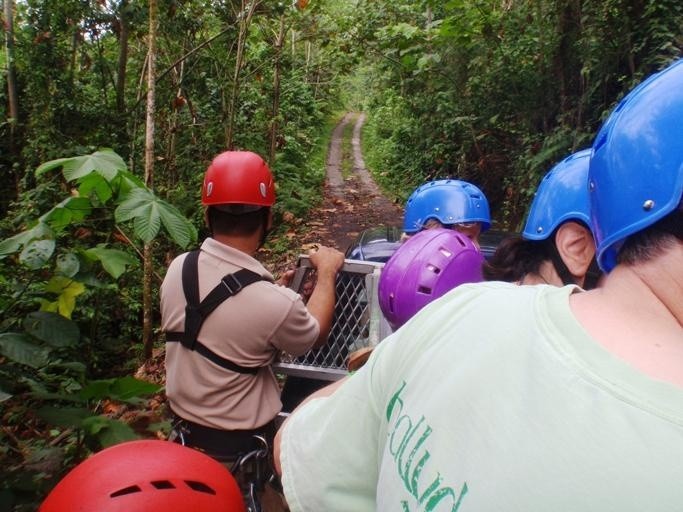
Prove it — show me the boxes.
[274,55,683,510]
[396,176,493,252]
[490,145,609,295]
[158,148,348,511]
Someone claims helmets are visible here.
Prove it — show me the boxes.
[198,147,278,207]
[399,175,493,236]
[518,147,599,249]
[586,59,683,278]
